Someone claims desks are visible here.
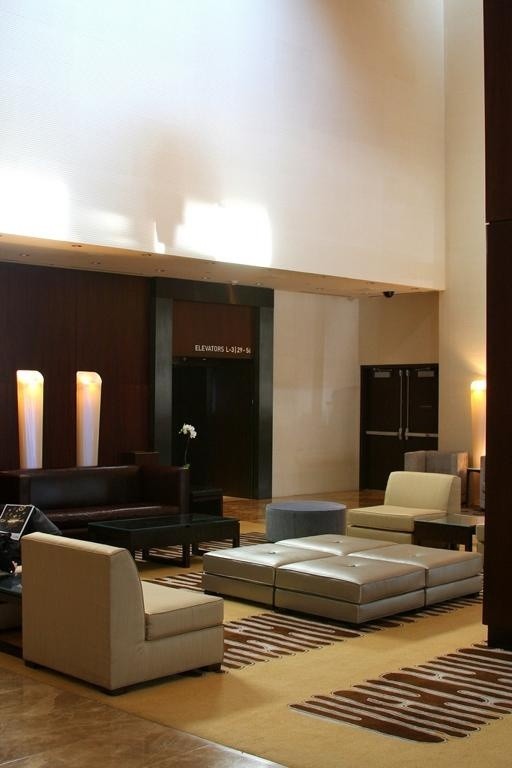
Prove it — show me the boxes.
[87,512,242,568]
[412,512,478,555]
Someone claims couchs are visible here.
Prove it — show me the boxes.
[0,462,191,529]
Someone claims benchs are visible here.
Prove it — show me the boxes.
[198,532,481,627]
[264,501,347,545]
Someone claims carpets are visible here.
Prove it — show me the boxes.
[0,519,512,768]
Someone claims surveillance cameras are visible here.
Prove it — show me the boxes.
[382,289,395,298]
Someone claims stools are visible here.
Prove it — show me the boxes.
[189,484,224,520]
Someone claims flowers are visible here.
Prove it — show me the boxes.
[177,423,198,464]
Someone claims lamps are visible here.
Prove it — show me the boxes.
[14,367,106,468]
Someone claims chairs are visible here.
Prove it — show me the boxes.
[347,450,468,546]
[19,528,228,696]
